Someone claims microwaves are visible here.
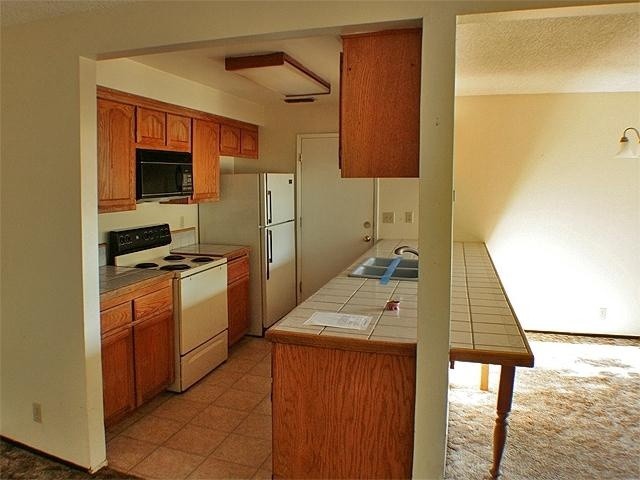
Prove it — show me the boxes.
[135,148,193,205]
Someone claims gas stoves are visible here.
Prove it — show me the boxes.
[106,224,228,392]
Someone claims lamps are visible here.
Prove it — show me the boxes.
[615,126,640,160]
[224,53,329,98]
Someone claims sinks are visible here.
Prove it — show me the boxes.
[348,255,419,283]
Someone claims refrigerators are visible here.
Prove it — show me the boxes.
[198,173,295,337]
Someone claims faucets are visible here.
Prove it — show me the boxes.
[394,245,418,256]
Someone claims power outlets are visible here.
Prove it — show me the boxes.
[31,403,45,423]
[406,212,414,223]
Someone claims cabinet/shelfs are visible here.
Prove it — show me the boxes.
[193,108,222,207]
[136,94,193,154]
[97,83,136,214]
[99,264,176,432]
[172,243,251,350]
[335,28,420,178]
[220,117,259,159]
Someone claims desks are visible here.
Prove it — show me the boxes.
[267,237,419,479]
[449,240,534,478]
